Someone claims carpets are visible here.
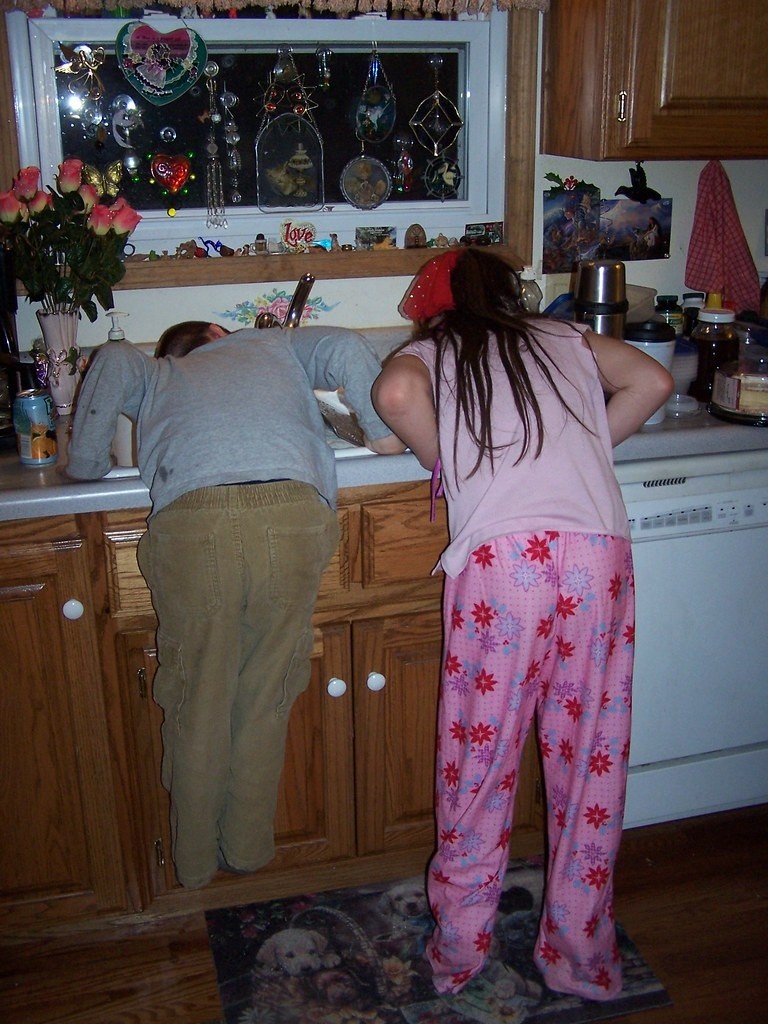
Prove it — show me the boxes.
[204,868,673,1024]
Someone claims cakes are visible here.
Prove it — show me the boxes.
[729,373,768,412]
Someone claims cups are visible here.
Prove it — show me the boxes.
[250,239,268,255]
[622,319,676,425]
[267,236,287,255]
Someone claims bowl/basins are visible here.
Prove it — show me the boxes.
[666,353,698,419]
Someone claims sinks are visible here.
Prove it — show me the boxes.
[97,414,349,479]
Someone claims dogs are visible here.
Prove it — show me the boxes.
[495,908,546,952]
[255,884,429,1007]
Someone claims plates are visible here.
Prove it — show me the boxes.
[705,401,768,428]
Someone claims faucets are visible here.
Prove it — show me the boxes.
[281,272,316,330]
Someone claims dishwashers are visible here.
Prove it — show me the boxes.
[612,450,768,831]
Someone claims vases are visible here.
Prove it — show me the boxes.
[36,303,86,416]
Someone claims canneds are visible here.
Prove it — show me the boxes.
[12,387,60,467]
[655,292,706,337]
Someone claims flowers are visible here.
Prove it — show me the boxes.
[0,159,142,321]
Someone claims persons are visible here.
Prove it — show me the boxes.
[370,248,676,1000]
[56,320,406,889]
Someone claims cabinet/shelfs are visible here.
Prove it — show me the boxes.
[539,0,768,164]
[0,472,549,951]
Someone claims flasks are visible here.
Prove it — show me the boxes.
[573,259,629,340]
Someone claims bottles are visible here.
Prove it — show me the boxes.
[515,266,543,315]
[688,308,740,403]
[655,296,683,336]
[713,345,768,417]
[680,293,706,337]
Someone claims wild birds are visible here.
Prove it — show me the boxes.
[614,165,662,205]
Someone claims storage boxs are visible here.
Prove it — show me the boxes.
[624,284,657,324]
